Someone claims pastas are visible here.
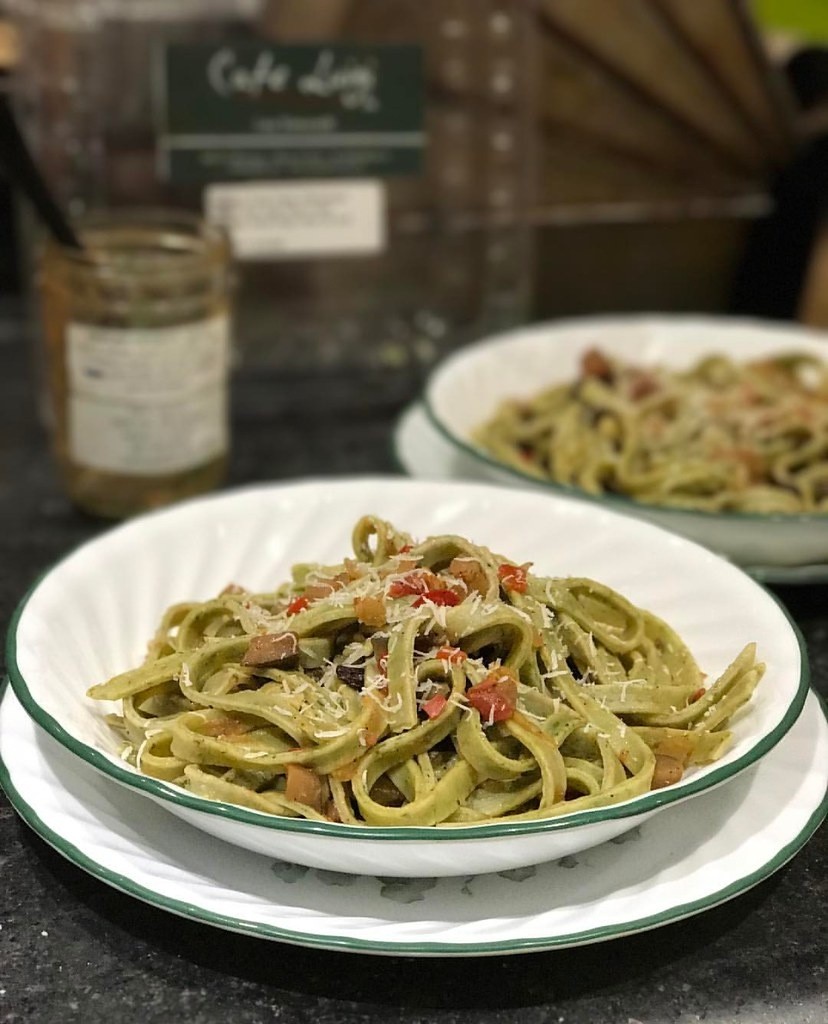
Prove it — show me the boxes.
[480,344,828,520]
[87,515,767,825]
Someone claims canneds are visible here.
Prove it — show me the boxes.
[36,209,234,516]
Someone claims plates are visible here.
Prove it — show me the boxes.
[0,686,828,958]
[393,401,828,580]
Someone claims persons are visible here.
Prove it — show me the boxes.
[726,47,828,328]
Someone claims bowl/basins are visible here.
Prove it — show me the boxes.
[6,475,811,877]
[420,307,828,562]
